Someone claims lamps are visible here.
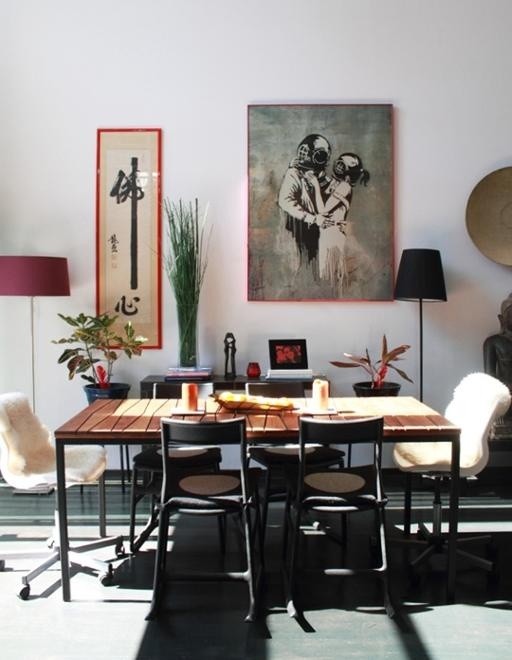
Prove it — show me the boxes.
[396,249,447,403]
[0,255,71,496]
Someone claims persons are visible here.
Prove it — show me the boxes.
[276,349,287,364]
[483,293,512,391]
[275,134,354,278]
[281,344,300,363]
[305,150,375,284]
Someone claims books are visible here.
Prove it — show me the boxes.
[164,375,211,382]
[166,365,213,376]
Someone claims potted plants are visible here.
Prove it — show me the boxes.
[50,311,151,405]
[163,196,214,368]
[329,335,414,398]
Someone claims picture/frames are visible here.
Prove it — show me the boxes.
[246,102,396,302]
[93,127,162,349]
[269,339,308,370]
